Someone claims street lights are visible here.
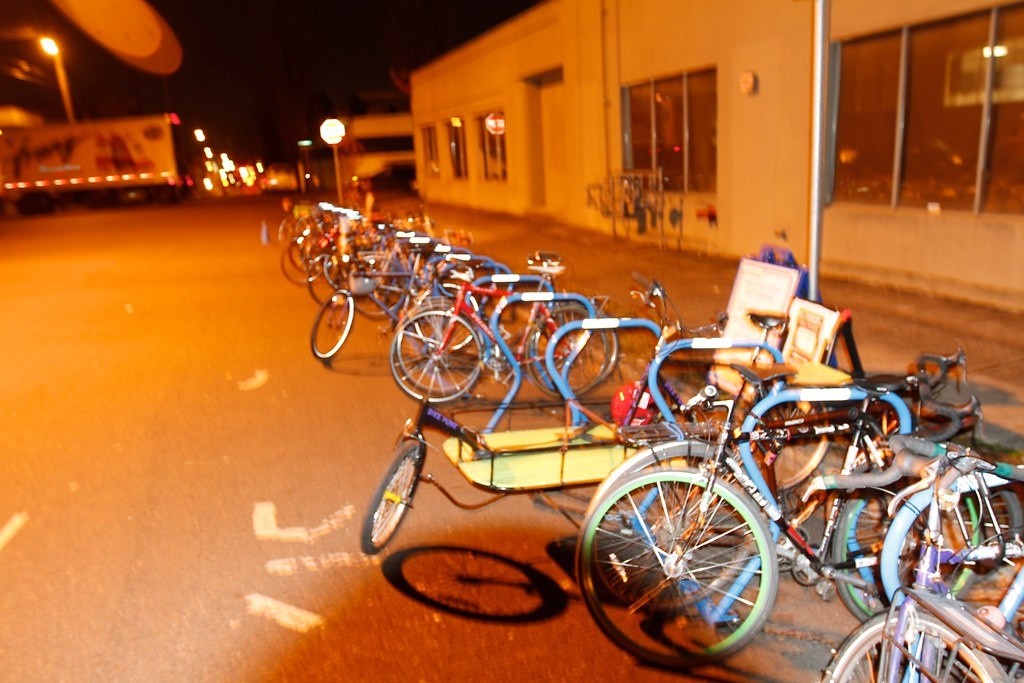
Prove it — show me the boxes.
[319,118,346,208]
[42,36,84,122]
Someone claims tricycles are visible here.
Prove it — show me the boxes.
[359,243,869,556]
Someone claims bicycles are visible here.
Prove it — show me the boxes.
[574,269,1024,683]
[278,196,620,404]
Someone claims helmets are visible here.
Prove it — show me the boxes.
[349,269,378,296]
